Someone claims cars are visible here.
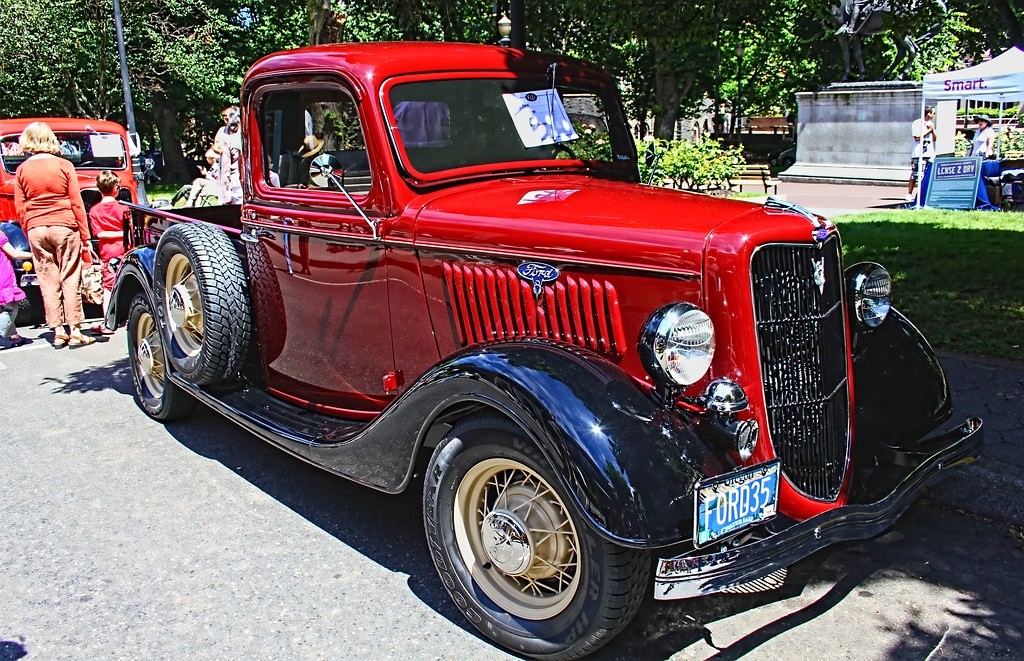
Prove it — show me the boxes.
[106,39,981,660]
[0,117,156,326]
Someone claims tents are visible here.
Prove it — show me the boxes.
[917,45,1024,207]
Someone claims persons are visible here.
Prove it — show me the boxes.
[969,114,994,158]
[906,106,937,201]
[89,169,135,324]
[637,121,650,137]
[393,101,451,147]
[169,106,314,207]
[14,122,97,349]
[3,141,24,156]
[60,140,77,155]
[0,231,33,347]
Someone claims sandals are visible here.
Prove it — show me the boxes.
[68,330,96,349]
[54,335,70,348]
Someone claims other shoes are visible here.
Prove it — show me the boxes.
[0,335,33,348]
[102,320,115,334]
[906,194,912,202]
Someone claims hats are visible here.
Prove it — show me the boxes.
[974,114,993,129]
[298,134,325,158]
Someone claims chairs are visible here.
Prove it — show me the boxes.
[999,159,1024,209]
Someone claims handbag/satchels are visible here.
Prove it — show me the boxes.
[79,246,105,306]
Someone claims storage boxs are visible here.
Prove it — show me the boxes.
[987,186,999,205]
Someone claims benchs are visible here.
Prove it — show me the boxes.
[288,147,478,192]
[726,165,783,194]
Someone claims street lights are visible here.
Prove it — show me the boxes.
[734,41,745,148]
